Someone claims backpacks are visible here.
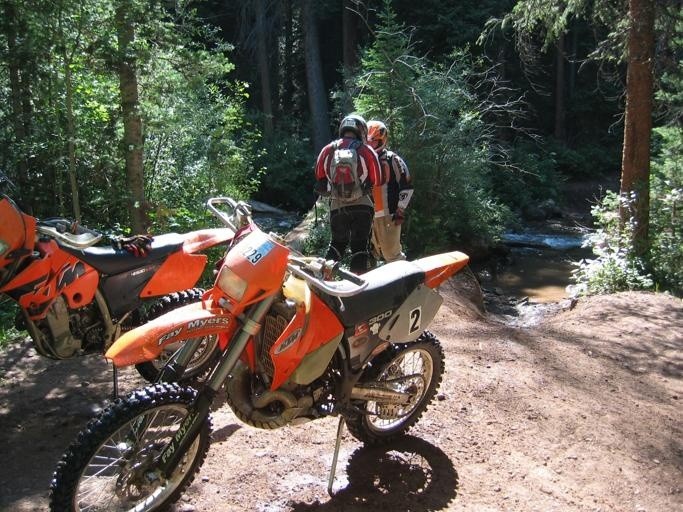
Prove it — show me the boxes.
[327,139,369,202]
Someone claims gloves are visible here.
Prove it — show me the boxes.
[392,208,404,225]
[112,234,152,258]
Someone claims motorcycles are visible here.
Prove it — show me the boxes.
[1,172,235,359]
[49,196,470,512]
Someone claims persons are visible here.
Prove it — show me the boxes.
[312,115,384,272]
[354,119,415,267]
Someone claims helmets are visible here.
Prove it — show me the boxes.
[368,121,387,153]
[339,115,368,141]
